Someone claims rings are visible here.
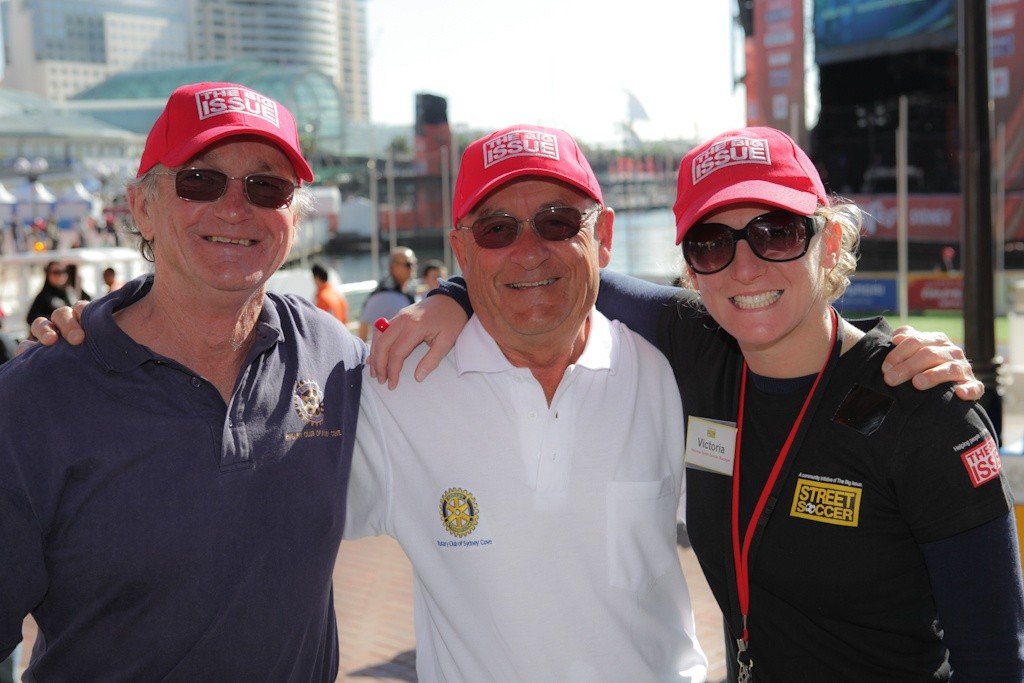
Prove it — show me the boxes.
[374,316,389,332]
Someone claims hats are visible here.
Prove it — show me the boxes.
[136,82,315,188]
[452,123,604,230]
[673,126,829,246]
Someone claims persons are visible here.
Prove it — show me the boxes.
[16,125,986,682]
[359,246,418,343]
[24,261,92,342]
[102,267,121,293]
[0,80,367,683]
[312,264,348,325]
[423,258,449,299]
[366,125,1024,683]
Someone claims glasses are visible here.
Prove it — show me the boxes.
[50,270,68,275]
[682,210,828,275]
[459,206,599,249]
[404,258,421,267]
[149,167,300,209]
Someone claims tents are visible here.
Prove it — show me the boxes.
[0,158,103,230]
